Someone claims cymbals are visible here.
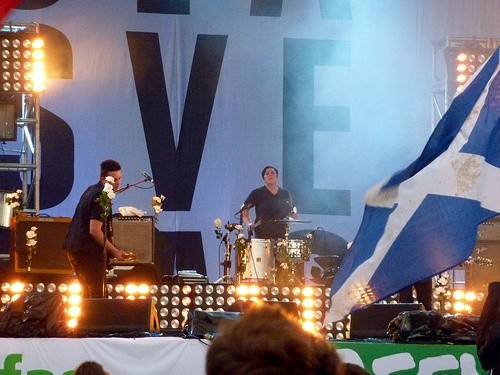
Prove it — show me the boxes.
[264,218,314,227]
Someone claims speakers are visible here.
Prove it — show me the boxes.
[10,215,155,274]
[77,298,160,333]
[350,304,426,338]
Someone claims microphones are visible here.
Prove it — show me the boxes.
[235,203,253,215]
[304,227,319,239]
[142,172,155,183]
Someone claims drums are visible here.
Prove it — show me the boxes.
[278,236,312,264]
[236,237,277,281]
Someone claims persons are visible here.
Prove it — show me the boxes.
[206,305,346,375]
[74,361,110,375]
[242,166,297,240]
[62,160,127,299]
[384,277,431,311]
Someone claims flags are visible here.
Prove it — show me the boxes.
[319,44,500,330]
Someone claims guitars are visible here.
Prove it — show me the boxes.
[102,251,140,271]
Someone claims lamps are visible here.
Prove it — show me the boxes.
[0,102,17,144]
[0,280,486,342]
[0,31,46,94]
[447,48,495,99]
